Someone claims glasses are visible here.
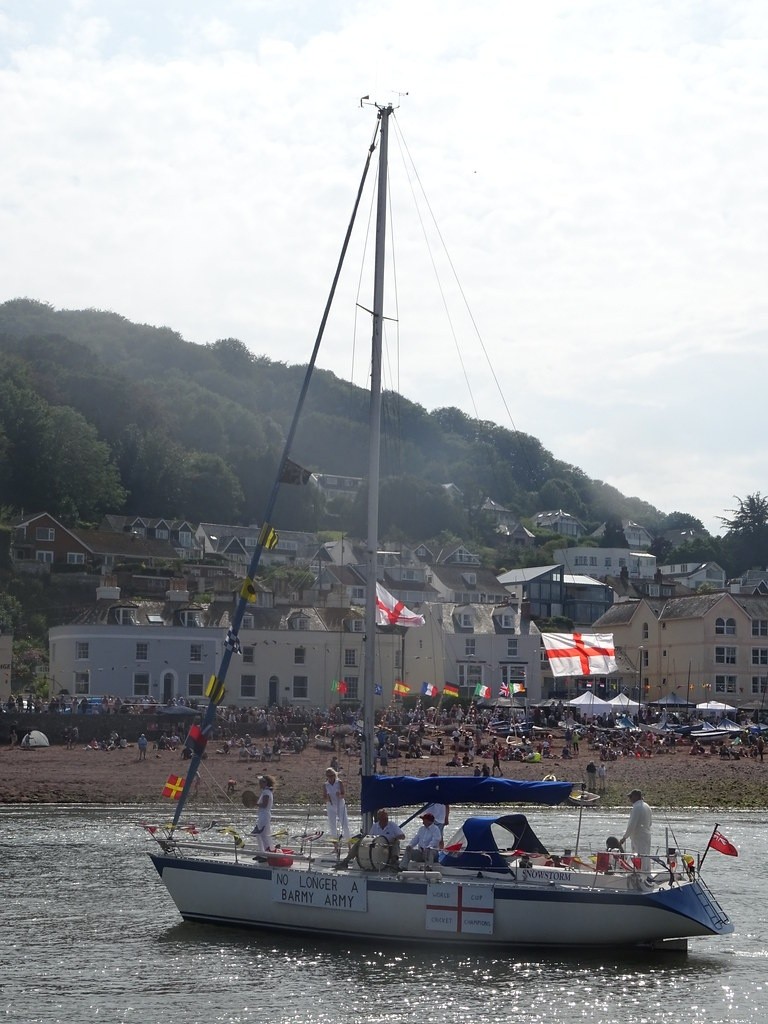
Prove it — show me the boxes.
[327,775,333,777]
[628,795,632,798]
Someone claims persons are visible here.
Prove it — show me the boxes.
[393,813,441,871]
[332,808,406,870]
[251,775,276,863]
[0,684,767,792]
[322,768,350,842]
[423,772,450,850]
[620,789,652,879]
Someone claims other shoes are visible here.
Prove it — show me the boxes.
[393,866,407,873]
[332,861,348,869]
[426,866,432,871]
[252,856,267,863]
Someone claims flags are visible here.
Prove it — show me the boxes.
[373,682,384,696]
[277,453,312,487]
[540,631,619,678]
[224,626,244,656]
[186,725,208,756]
[376,582,426,627]
[498,681,526,699]
[256,522,280,551]
[330,678,347,695]
[475,682,492,699]
[392,680,411,697]
[163,775,185,799]
[709,830,739,857]
[442,680,459,697]
[420,681,438,697]
[205,674,228,706]
[239,574,258,604]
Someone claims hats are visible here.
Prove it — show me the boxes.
[420,813,434,822]
[626,789,641,796]
[257,776,266,781]
[464,752,468,755]
[457,758,461,760]
[475,764,479,768]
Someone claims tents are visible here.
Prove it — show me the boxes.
[531,698,577,722]
[696,700,736,720]
[567,692,610,722]
[607,693,644,717]
[737,700,767,716]
[649,692,696,719]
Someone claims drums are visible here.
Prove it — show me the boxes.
[357,834,391,871]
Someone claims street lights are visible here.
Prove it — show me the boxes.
[638,645,643,724]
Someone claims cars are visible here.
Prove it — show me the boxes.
[58,697,110,715]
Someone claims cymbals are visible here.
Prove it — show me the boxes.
[242,790,257,809]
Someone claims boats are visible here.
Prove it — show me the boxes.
[147,774,738,948]
[310,713,768,751]
